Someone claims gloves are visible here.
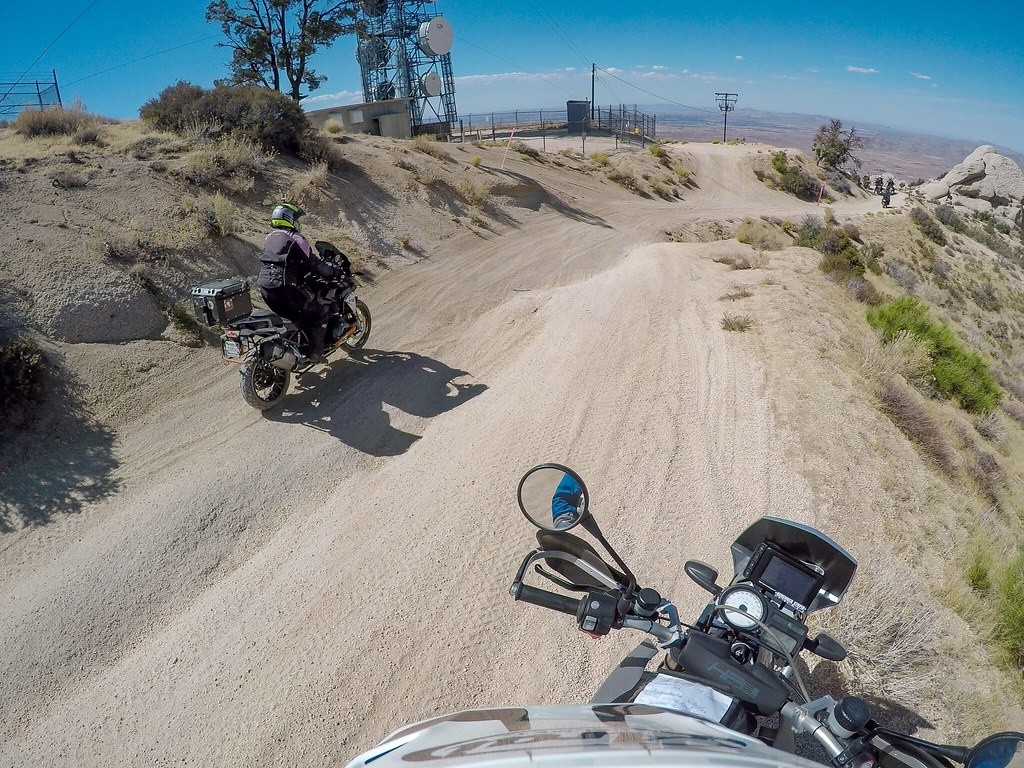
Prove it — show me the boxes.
[336,269,346,282]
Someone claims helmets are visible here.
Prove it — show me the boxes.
[270,203,306,232]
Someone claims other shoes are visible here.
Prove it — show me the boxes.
[309,353,328,363]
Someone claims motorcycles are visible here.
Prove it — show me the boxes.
[862,173,897,209]
[191,241,371,410]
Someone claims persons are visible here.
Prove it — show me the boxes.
[882,186,891,202]
[552,473,583,529]
[863,173,869,186]
[257,203,344,364]
[886,177,894,189]
[873,174,883,194]
